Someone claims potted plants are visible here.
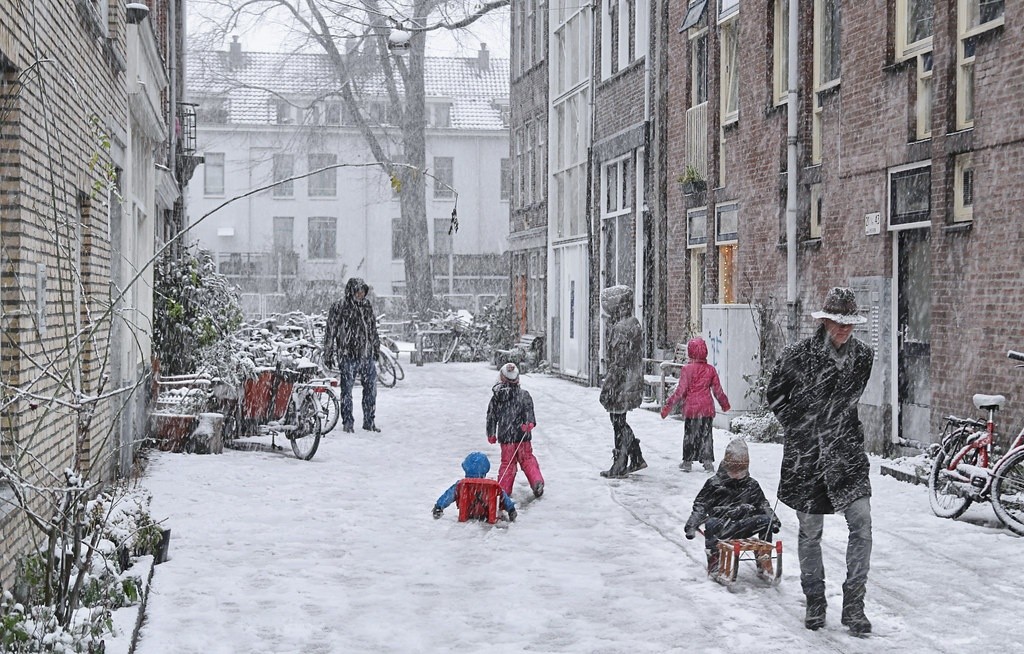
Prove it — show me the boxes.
[677,165,707,194]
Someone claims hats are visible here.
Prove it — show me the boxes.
[720,438,749,471]
[500,363,519,384]
[811,287,869,324]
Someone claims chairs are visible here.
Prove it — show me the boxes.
[458,477,503,522]
[717,535,777,584]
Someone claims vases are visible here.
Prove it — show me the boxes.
[137,528,172,565]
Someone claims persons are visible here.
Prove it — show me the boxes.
[767,287,876,633]
[324,278,381,433]
[685,437,781,576]
[486,362,545,498]
[662,338,731,472]
[432,452,517,521]
[599,285,648,479]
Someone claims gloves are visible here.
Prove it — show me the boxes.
[686,526,696,539]
[433,504,443,519]
[373,354,380,361]
[509,508,517,521]
[721,402,731,412]
[325,359,336,371]
[661,405,671,419]
[770,515,781,534]
[520,423,535,431]
[488,435,496,444]
[599,388,613,409]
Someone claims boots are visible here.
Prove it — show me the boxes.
[599,448,629,479]
[842,583,871,638]
[626,439,648,474]
[757,550,773,577]
[802,581,828,631]
[708,552,719,574]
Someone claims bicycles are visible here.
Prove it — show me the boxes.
[927,349,1024,537]
[216,298,510,461]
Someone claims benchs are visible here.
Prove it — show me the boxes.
[493,331,544,371]
[643,344,689,411]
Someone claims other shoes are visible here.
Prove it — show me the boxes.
[679,460,692,470]
[702,461,715,472]
[343,424,354,433]
[362,424,381,432]
[533,481,544,498]
